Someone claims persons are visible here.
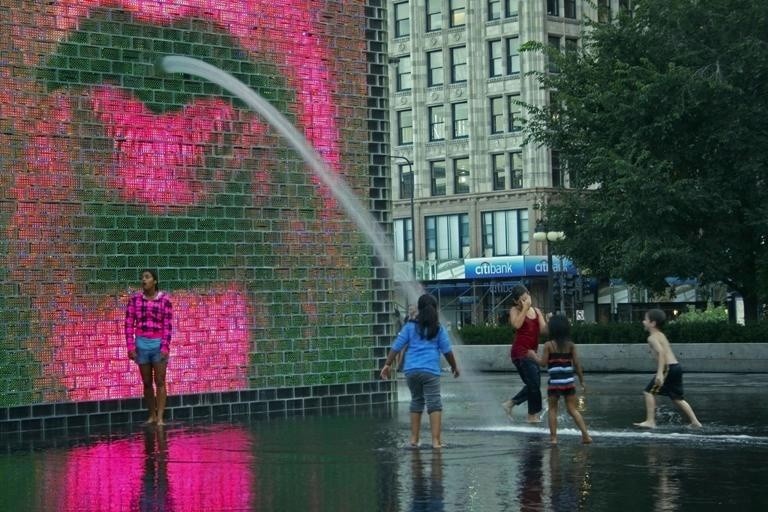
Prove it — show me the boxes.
[500,285,553,424]
[527,314,593,444]
[632,308,702,429]
[380,294,460,448]
[124,270,172,426]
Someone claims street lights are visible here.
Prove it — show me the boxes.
[532,228,567,317]
